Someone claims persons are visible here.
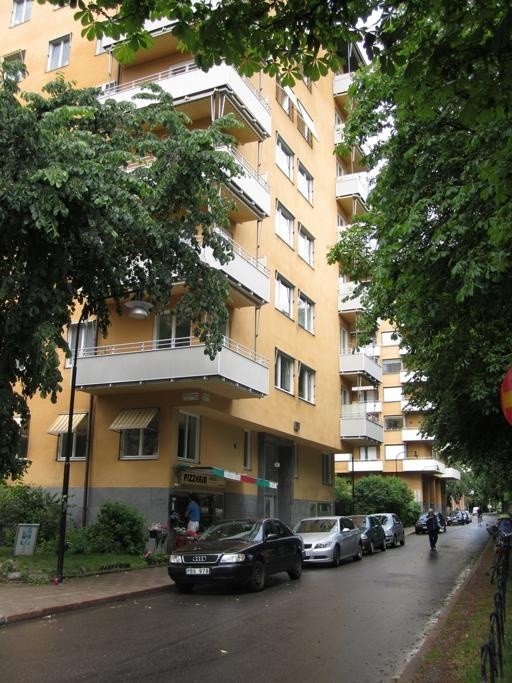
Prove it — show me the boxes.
[184,494,201,535]
[422,508,442,550]
[477,505,483,525]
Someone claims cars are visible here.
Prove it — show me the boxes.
[447,510,472,526]
[167,513,405,594]
[415,512,446,534]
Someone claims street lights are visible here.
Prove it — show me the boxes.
[396,450,419,480]
[56,288,155,583]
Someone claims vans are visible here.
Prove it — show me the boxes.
[472,506,481,516]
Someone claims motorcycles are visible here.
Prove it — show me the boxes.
[495,517,512,546]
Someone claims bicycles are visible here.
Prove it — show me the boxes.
[484,544,505,584]
[477,515,483,527]
